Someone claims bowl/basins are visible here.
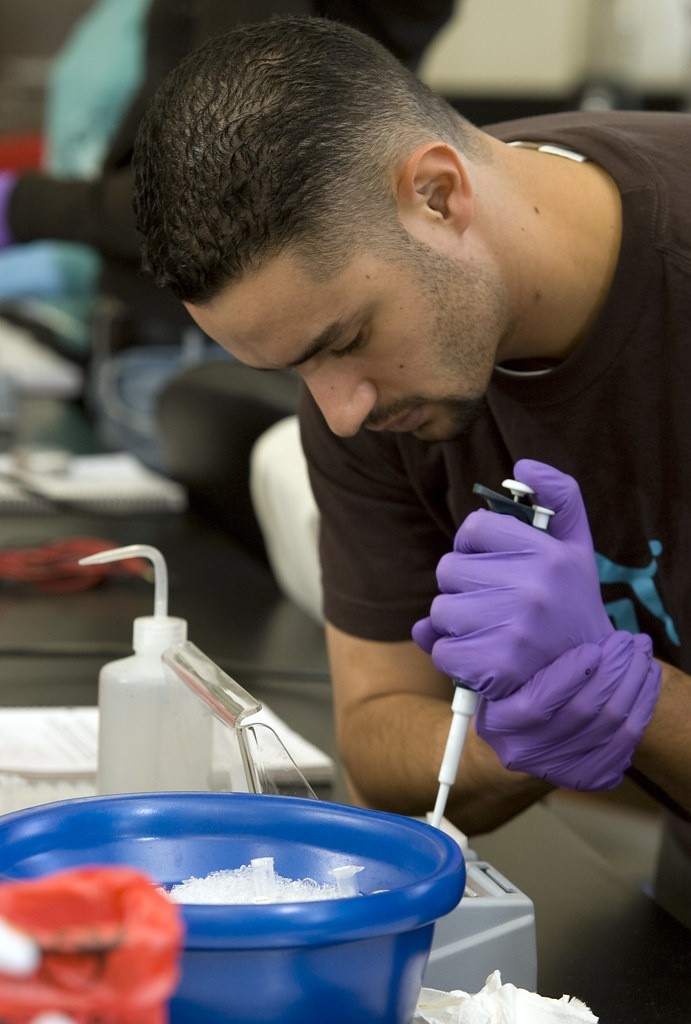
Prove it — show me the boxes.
[0,790,467,1024]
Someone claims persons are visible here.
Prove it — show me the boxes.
[128,14,691,838]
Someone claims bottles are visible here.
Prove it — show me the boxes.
[80,544,212,796]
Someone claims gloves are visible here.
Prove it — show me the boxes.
[411,459,617,700]
[473,630,661,793]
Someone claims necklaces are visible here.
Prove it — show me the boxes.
[491,140,589,379]
